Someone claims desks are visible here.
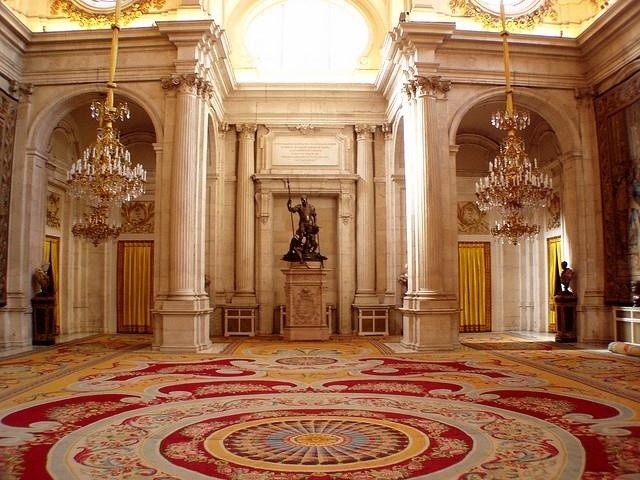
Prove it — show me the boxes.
[352,304,396,336]
[219,304,260,337]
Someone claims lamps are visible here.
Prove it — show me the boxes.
[65,0,148,246]
[475,0,560,244]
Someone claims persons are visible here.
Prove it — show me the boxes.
[287,194,317,253]
